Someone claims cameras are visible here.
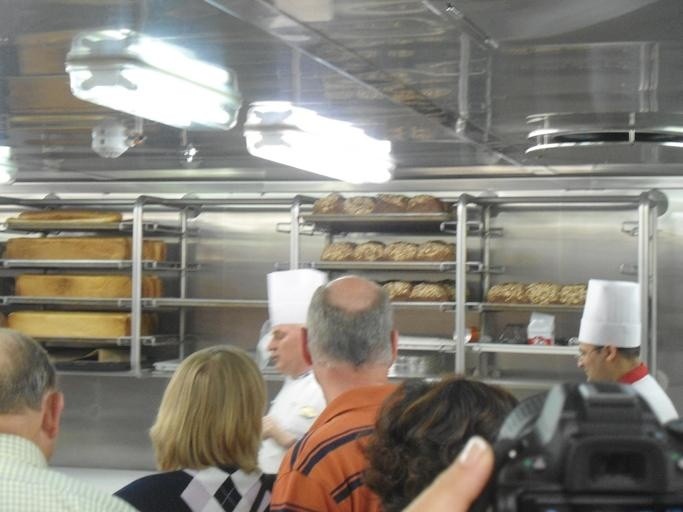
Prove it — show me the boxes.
[488,380,683,512]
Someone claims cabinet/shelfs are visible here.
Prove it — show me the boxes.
[135,192,293,381]
[460,189,666,380]
[0,195,139,382]
[296,198,461,380]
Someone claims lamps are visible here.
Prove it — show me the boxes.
[244,103,395,187]
[1,142,16,184]
[62,61,241,134]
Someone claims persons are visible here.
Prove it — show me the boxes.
[0,328,138,511]
[575,342,679,422]
[400,433,496,511]
[108,344,275,511]
[355,371,524,511]
[256,323,328,475]
[270,273,408,510]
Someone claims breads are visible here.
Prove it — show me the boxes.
[3,237,166,263]
[382,278,471,303]
[11,273,163,298]
[321,239,456,262]
[5,309,155,337]
[5,210,123,228]
[310,191,448,214]
[486,282,586,305]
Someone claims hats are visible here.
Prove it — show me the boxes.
[266,268,328,328]
[578,279,641,348]
